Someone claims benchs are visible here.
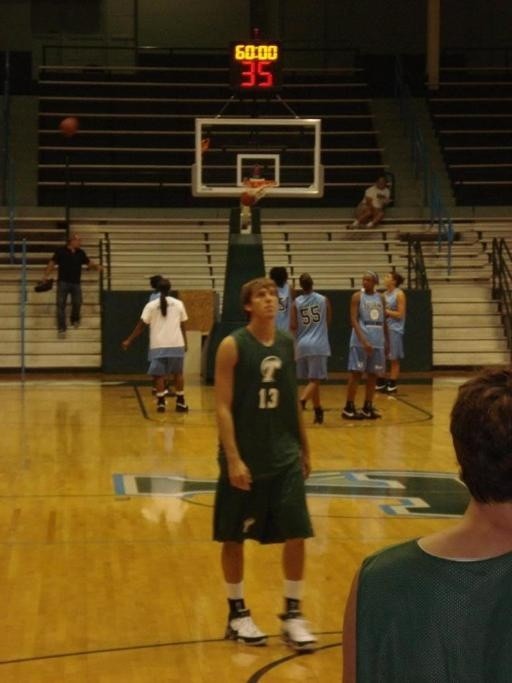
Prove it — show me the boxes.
[1,223,512,372]
[420,65,512,209]
[37,46,396,208]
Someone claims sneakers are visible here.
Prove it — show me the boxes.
[359,408,382,420]
[276,609,320,652]
[176,402,189,412]
[312,410,324,427]
[375,383,400,394]
[341,408,365,420]
[156,400,168,413]
[223,607,269,647]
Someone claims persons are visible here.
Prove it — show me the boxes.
[345,176,394,230]
[212,276,320,653]
[343,368,511,681]
[122,278,193,413]
[41,233,103,338]
[341,269,391,420]
[373,270,407,393]
[146,273,180,397]
[288,272,333,424]
[268,265,298,337]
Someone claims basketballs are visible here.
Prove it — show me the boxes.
[62,116,78,135]
[241,191,257,206]
